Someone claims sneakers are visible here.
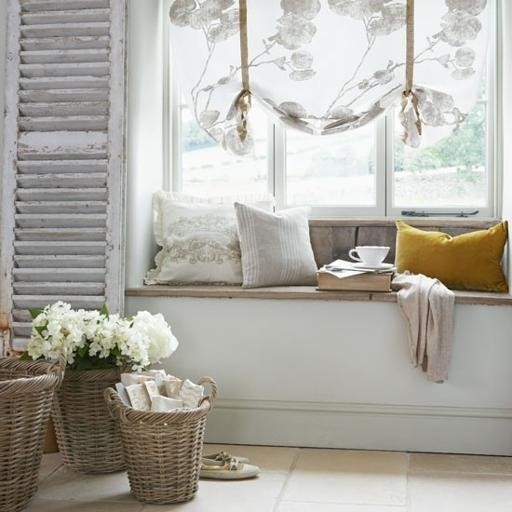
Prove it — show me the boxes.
[199,451,261,481]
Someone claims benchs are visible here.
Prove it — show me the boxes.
[125,217,512,458]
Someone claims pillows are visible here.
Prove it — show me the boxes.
[391,218,508,293]
[234,201,318,289]
[143,190,275,287]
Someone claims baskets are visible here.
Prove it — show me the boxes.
[48,366,137,478]
[104,373,218,506]
[0,346,71,512]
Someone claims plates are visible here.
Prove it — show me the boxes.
[352,263,393,269]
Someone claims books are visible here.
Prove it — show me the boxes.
[316,269,391,293]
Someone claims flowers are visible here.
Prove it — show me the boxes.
[20,301,179,374]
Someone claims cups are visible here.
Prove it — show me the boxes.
[348,246,390,265]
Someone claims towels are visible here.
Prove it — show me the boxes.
[391,270,456,385]
